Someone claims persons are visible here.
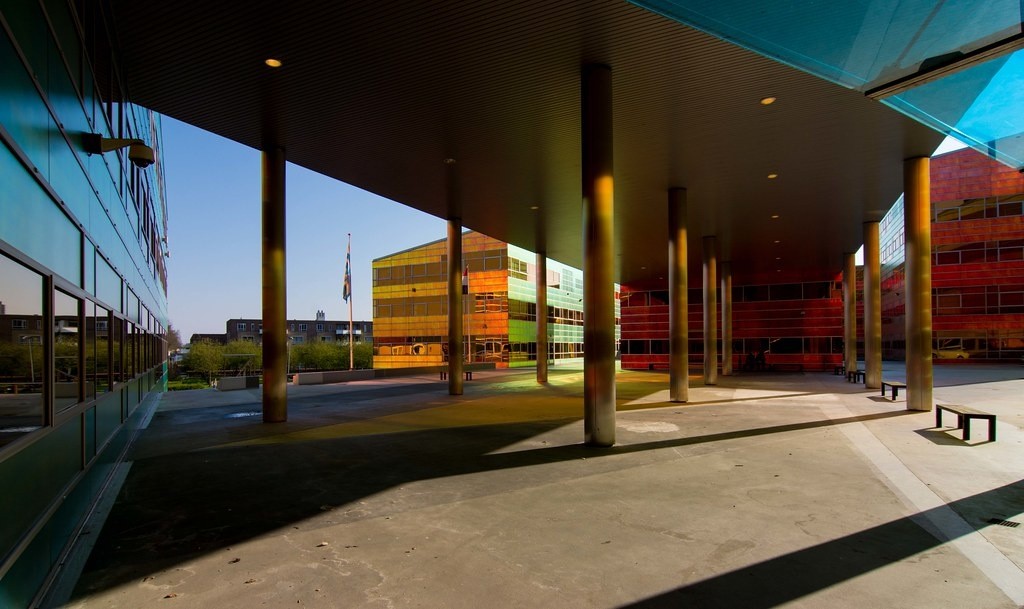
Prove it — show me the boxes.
[744,351,757,371]
[756,350,765,371]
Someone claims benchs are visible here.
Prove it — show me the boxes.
[766,362,803,374]
[848,370,865,384]
[879,381,907,400]
[835,365,846,375]
[935,403,996,443]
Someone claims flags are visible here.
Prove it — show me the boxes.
[342,243,351,303]
[462,264,468,294]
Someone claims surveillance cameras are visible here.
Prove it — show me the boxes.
[128,144,157,170]
